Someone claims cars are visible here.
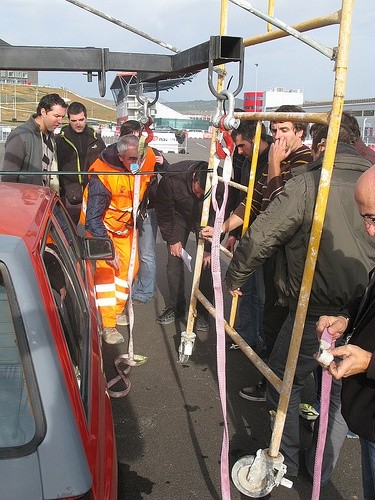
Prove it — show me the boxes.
[0,181,119,500]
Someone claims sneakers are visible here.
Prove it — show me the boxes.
[196,311,211,331]
[102,327,125,344]
[239,380,267,401]
[116,315,129,325]
[156,307,185,325]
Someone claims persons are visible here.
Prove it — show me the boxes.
[56,101,107,229]
[154,160,233,331]
[109,121,113,130]
[1,93,69,198]
[97,125,103,130]
[77,120,171,344]
[199,105,375,500]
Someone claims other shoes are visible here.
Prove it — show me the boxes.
[299,404,318,420]
[346,429,359,439]
[132,299,144,305]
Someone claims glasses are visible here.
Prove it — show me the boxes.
[360,217,375,225]
